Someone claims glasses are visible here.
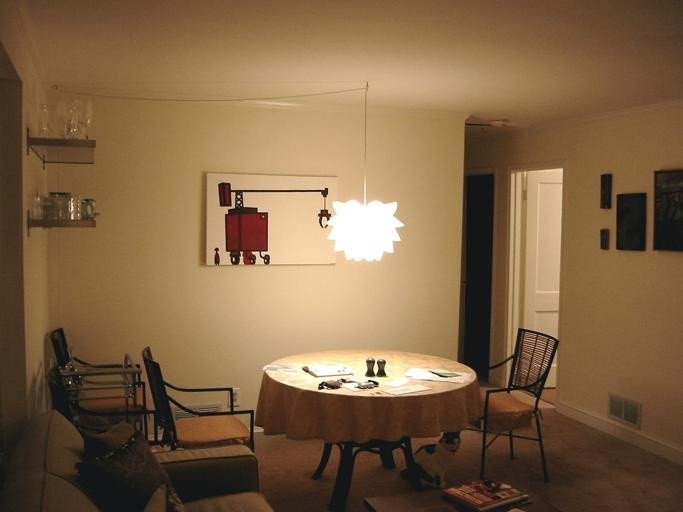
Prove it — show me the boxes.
[325,200,404,263]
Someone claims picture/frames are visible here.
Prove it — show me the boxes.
[362,485,529,512]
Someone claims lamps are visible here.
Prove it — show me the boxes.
[140,346,255,451]
[49,328,142,388]
[45,366,147,440]
[477,328,559,484]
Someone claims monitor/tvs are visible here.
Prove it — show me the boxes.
[440,476,532,511]
[302,363,353,378]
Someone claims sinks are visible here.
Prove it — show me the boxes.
[0,408,274,512]
